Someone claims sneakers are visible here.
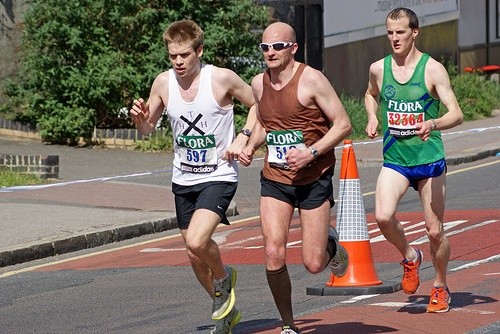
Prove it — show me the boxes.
[426,286,453,313]
[211,266,238,320]
[211,308,241,334]
[328,226,349,278]
[401,246,423,296]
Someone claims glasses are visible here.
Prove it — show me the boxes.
[259,42,292,51]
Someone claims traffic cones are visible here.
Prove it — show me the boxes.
[305,139,404,297]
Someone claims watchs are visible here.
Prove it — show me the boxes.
[308,145,319,161]
[239,129,252,138]
[430,119,436,130]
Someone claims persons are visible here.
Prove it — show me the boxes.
[130,18,259,334]
[236,22,351,334]
[360,7,464,316]
